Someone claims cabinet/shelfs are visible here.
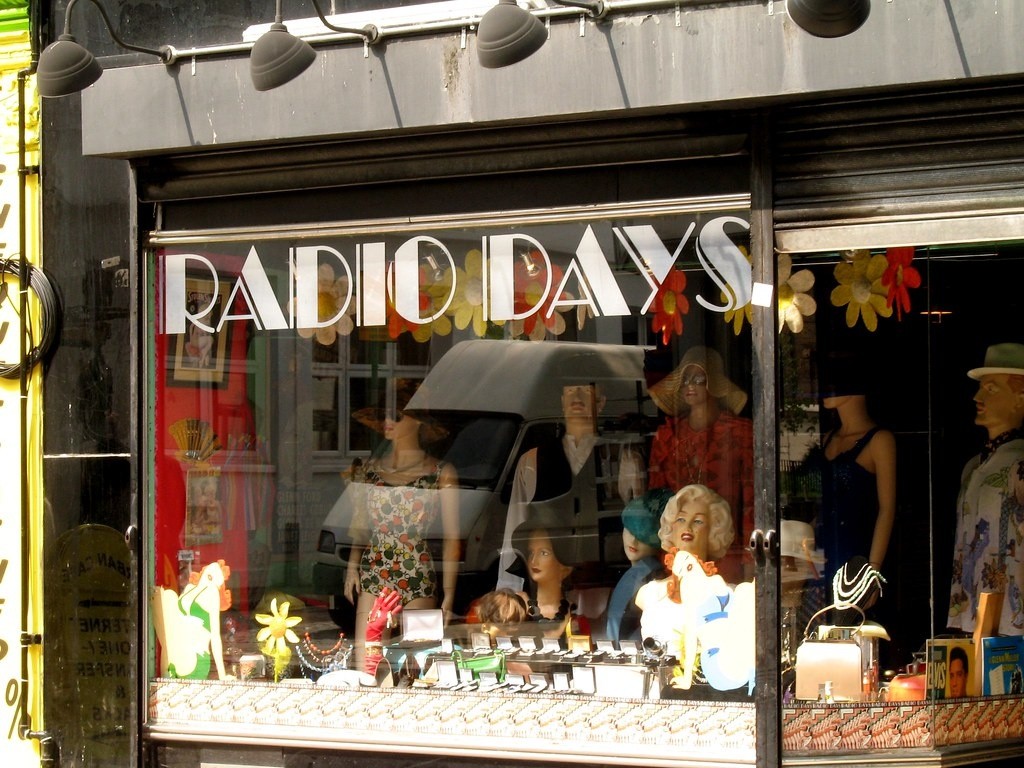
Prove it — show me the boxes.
[228,254,715,618]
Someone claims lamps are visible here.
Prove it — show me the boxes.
[476,0,609,68]
[36,0,178,99]
[249,0,383,91]
[784,0,872,39]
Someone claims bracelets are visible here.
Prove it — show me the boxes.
[867,561,882,565]
[476,647,486,651]
[414,637,428,641]
[363,640,384,656]
[366,606,380,623]
[387,610,398,628]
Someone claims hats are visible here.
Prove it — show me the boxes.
[967,344,1024,381]
[511,508,588,568]
[351,378,450,445]
[646,345,748,417]
[621,488,674,551]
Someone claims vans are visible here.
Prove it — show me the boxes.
[310,338,668,637]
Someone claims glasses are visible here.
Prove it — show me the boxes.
[680,372,706,386]
[377,410,404,422]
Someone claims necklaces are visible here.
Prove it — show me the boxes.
[683,414,705,484]
[832,562,887,610]
[377,454,426,474]
[295,631,353,684]
[529,598,570,621]
[497,644,512,651]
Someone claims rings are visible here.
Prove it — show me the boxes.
[379,591,385,597]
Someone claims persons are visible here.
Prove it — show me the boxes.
[945,340,1024,638]
[949,646,968,698]
[496,355,647,620]
[512,510,576,622]
[648,344,754,582]
[782,395,898,624]
[343,378,460,672]
[633,483,731,659]
[475,587,527,648]
[606,489,673,649]
